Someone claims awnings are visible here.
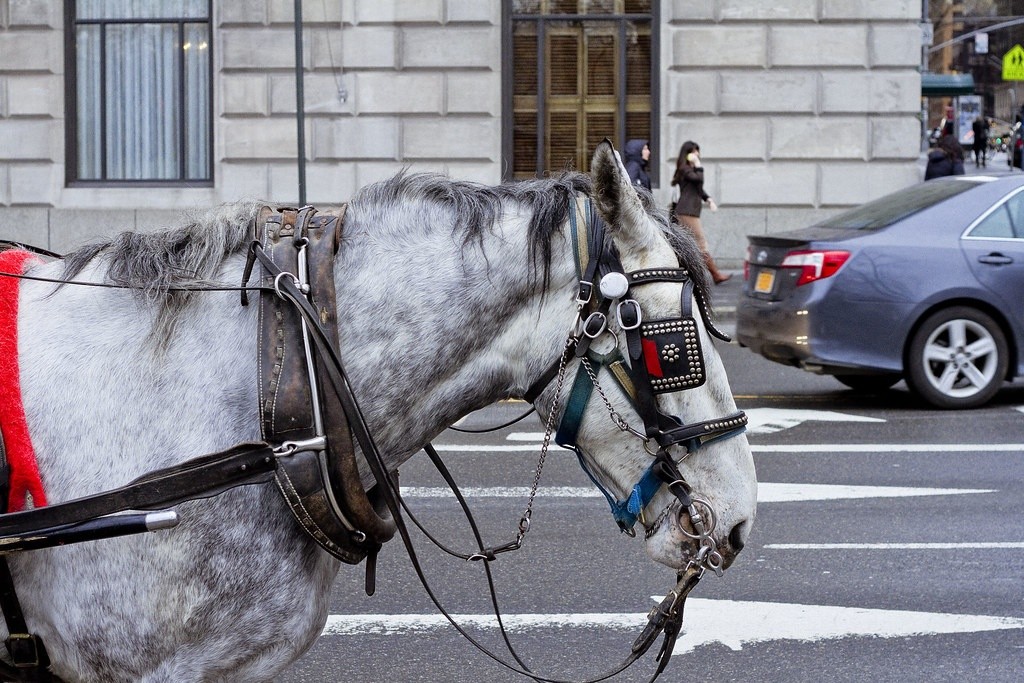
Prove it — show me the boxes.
[921,72,976,97]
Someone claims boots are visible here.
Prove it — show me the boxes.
[702,253,732,284]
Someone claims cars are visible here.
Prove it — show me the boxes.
[733,170,1024,410]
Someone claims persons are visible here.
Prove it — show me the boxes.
[669,140,734,287]
[925,134,965,181]
[972,115,990,168]
[624,137,652,191]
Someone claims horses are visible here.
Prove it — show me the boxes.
[0,137,757,683]
